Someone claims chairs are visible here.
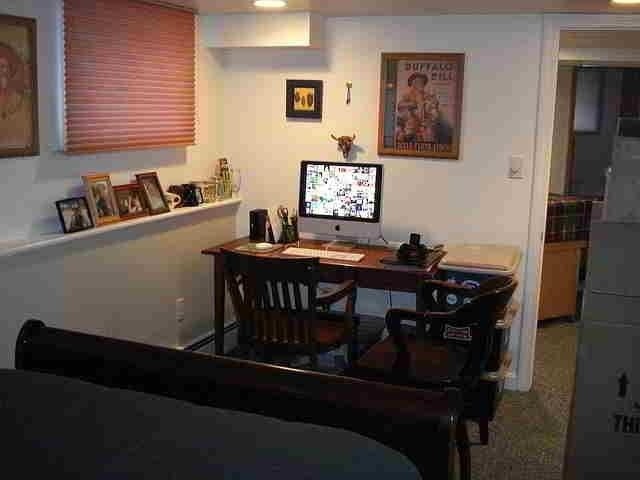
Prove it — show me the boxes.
[220,247,360,369]
[373,276,519,443]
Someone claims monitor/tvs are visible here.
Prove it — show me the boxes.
[298,160,383,223]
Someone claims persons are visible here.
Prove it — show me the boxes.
[395,72,440,150]
[68,204,86,232]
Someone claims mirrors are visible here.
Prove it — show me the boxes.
[574,71,604,136]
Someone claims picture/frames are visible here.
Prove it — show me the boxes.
[286,80,322,119]
[0,14,40,158]
[54,172,170,234]
[378,52,465,160]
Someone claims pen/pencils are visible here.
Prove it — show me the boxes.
[279,205,297,243]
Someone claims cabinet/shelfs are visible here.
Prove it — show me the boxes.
[538,240,587,322]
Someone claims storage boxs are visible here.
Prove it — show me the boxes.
[438,245,522,421]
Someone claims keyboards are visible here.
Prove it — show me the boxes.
[283,246,365,262]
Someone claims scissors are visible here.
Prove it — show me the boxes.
[277,208,288,225]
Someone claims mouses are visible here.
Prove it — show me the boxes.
[255,241,274,250]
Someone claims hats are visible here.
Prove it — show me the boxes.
[408,73,427,87]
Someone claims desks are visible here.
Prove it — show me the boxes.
[201,235,447,336]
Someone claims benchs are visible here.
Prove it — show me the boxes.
[0,369,422,480]
[15,319,471,480]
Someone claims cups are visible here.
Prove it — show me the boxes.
[283,225,297,243]
[164,176,233,211]
